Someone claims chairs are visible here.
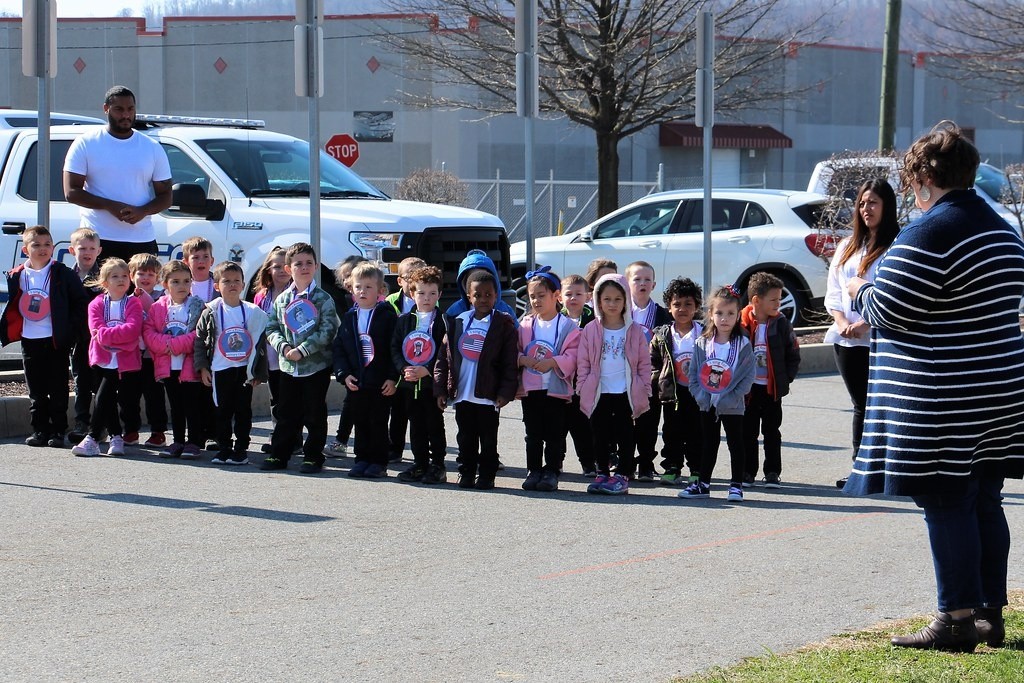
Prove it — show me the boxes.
[686,207,728,234]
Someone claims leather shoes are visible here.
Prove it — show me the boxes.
[974,606,1005,648]
[891,612,980,653]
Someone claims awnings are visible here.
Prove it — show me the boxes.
[659,123,793,148]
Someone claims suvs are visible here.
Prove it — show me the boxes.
[0,118,515,325]
[807,157,1024,237]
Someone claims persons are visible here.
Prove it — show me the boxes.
[414,342,422,357]
[822,178,901,490]
[845,119,1024,653]
[294,308,318,334]
[0,225,802,502]
[707,372,720,389]
[63,85,174,294]
[228,334,242,350]
[533,348,546,361]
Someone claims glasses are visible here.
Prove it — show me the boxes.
[400,276,413,283]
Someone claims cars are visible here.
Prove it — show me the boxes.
[510,187,855,329]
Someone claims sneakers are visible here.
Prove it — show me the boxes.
[742,475,756,487]
[727,481,744,501]
[660,467,682,484]
[637,466,655,482]
[678,480,710,498]
[26,424,629,496]
[762,472,781,488]
[688,475,699,483]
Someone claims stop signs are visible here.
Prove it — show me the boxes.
[324,134,359,167]
